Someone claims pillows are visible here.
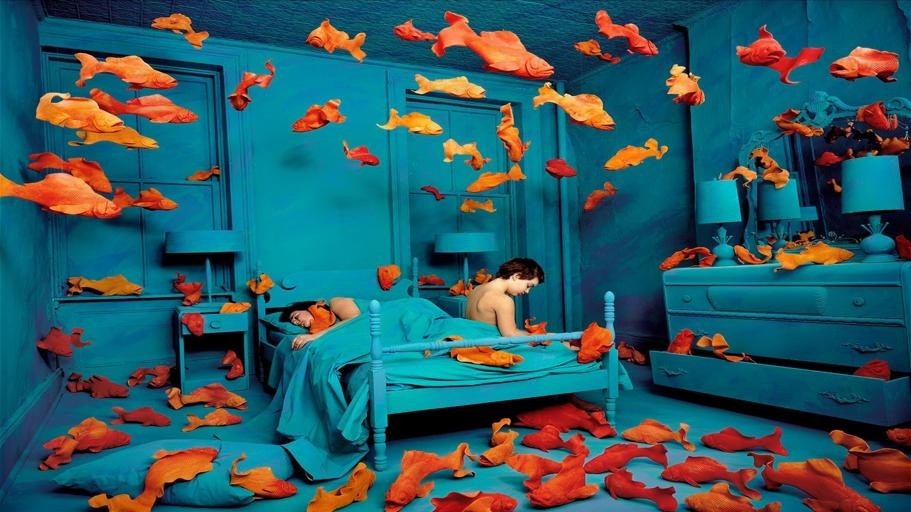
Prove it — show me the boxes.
[259,312,309,335]
[50,438,298,507]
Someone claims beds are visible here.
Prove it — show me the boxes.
[256,257,619,471]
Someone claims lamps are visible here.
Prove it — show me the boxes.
[435,233,498,297]
[841,156,905,263]
[166,229,246,308]
[695,177,742,266]
[797,207,819,234]
[758,178,801,252]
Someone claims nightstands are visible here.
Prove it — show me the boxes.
[175,307,250,394]
[434,296,467,318]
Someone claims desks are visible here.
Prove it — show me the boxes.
[649,262,911,427]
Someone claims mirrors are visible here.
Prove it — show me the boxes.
[738,90,911,261]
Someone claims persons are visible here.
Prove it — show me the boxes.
[278,297,363,352]
[465,257,581,351]
